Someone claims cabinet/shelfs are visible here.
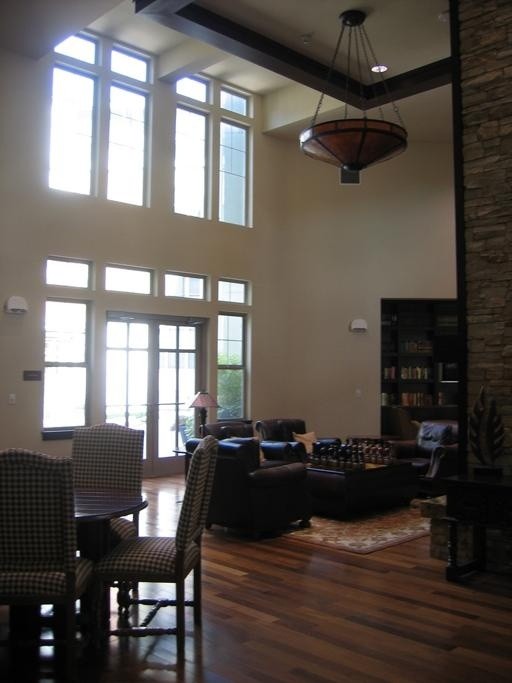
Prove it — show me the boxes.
[444,472,512,581]
[381,298,435,411]
[436,300,464,408]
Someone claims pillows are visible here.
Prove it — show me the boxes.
[416,421,453,456]
[292,431,317,454]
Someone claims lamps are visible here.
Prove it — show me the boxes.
[349,316,369,333]
[5,296,28,316]
[300,9,410,178]
[188,391,222,436]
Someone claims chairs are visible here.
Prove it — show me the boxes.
[199,421,294,467]
[387,418,460,495]
[0,448,101,682]
[254,419,342,461]
[185,438,311,540]
[70,423,144,615]
[92,436,220,659]
[381,406,418,432]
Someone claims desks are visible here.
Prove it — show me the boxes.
[71,489,148,670]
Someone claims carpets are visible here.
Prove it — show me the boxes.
[279,496,431,555]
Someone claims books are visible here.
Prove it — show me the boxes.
[435,314,458,334]
[400,339,432,352]
[383,366,429,381]
[381,391,444,406]
